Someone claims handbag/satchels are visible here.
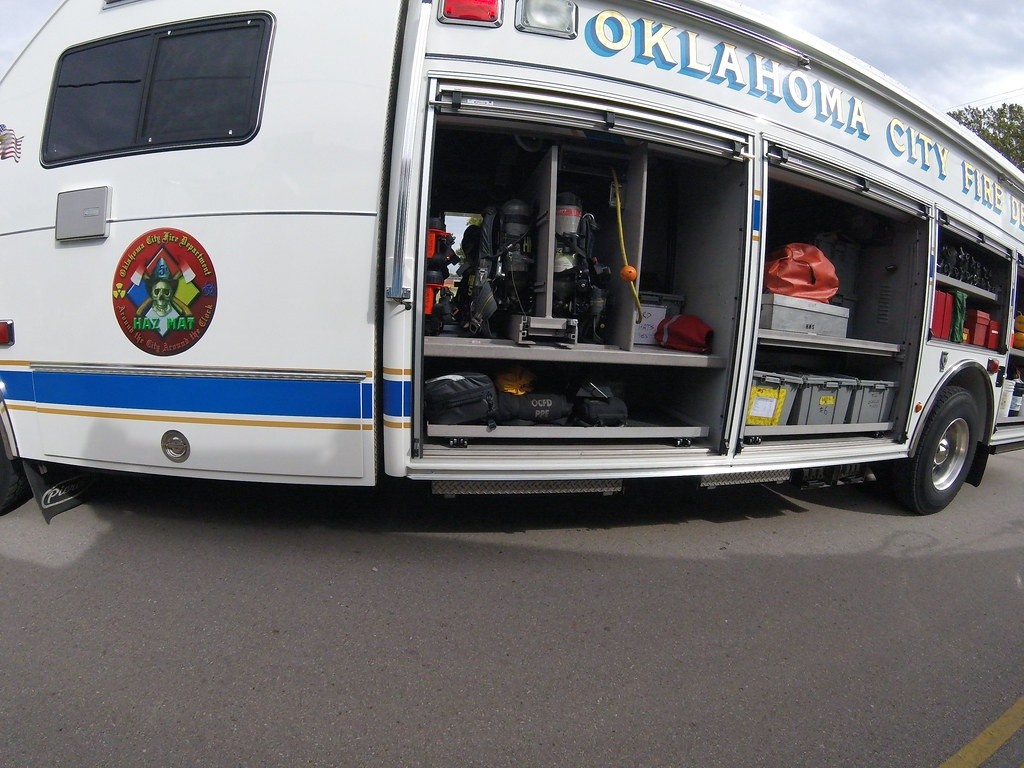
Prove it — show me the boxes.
[423,370,498,430]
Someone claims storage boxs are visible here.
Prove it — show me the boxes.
[985,320,1002,351]
[965,323,988,346]
[949,291,968,343]
[772,368,858,425]
[632,304,668,345]
[964,310,992,326]
[640,291,685,317]
[963,328,972,344]
[932,290,954,339]
[759,293,850,337]
[822,371,900,423]
[746,370,803,426]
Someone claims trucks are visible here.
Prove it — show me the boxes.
[0,0,1024,523]
